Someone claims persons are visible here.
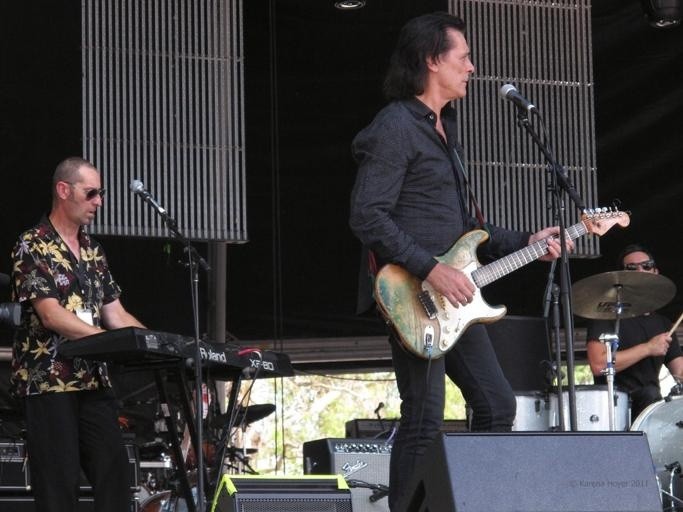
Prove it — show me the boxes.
[584,241,682,432]
[347,10,571,510]
[7,156,146,510]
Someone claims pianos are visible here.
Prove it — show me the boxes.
[57,327,295,381]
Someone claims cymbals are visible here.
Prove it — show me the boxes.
[560,270,677,321]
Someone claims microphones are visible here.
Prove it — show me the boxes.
[375,403,383,413]
[130,180,166,215]
[500,84,538,113]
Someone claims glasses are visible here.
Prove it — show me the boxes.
[68,183,106,201]
[627,259,655,271]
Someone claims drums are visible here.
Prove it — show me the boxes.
[465,384,631,432]
[632,394,682,512]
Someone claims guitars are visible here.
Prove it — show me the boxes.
[373,205,631,361]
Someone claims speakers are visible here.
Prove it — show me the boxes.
[388,430,662,512]
[303,438,394,512]
